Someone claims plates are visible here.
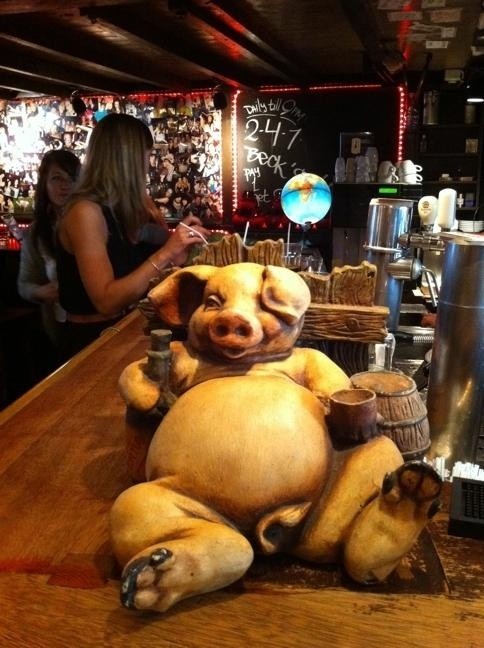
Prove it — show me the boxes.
[458,219,483,233]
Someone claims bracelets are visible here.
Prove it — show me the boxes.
[148,259,161,274]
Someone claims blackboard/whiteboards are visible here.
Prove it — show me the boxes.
[236,87,399,217]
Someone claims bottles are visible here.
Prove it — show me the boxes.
[422,89,441,126]
[419,133,428,152]
[463,96,478,124]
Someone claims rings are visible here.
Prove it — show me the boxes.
[189,232,194,237]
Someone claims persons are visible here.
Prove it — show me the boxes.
[55,113,212,344]
[17,149,80,346]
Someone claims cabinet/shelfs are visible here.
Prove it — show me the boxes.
[404,85,484,220]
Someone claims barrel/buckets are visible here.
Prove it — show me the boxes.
[349,370,432,458]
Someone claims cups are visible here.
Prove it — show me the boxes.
[328,387,378,448]
[335,146,377,183]
[367,330,396,370]
[464,138,479,153]
[304,256,323,272]
[377,160,424,183]
[279,242,302,270]
